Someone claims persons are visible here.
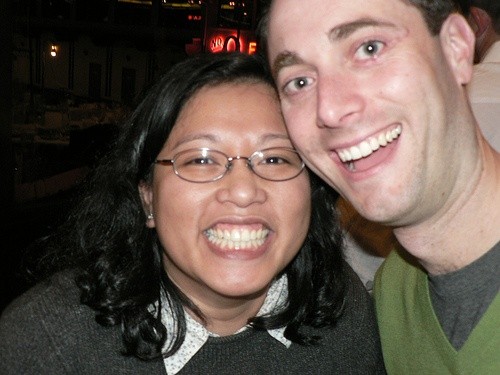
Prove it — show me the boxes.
[0,52,387,375]
[258,1,500,375]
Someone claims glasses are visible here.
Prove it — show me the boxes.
[155,147,308,183]
